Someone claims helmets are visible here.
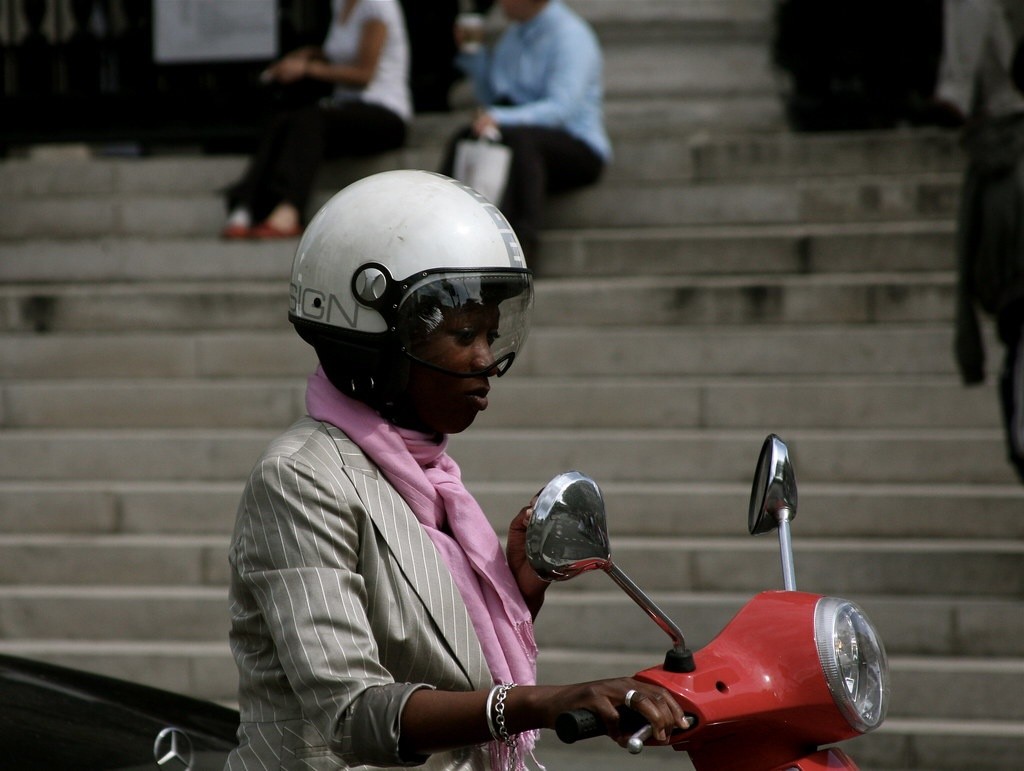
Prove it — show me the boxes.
[288,169,535,414]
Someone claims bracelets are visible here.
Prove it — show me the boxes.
[486,683,522,749]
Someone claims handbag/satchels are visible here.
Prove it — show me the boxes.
[452,123,513,209]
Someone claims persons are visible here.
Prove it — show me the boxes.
[935,0,1022,491]
[440,0,614,255]
[223,170,692,771]
[219,0,409,238]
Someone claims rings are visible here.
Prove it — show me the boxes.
[626,689,636,704]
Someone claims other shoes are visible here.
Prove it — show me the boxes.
[221,209,301,240]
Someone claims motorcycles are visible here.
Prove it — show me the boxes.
[1,433,892,770]
